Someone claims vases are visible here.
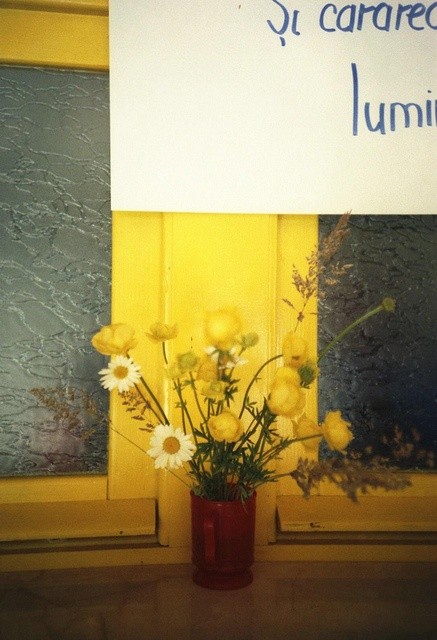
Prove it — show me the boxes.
[191,481,258,591]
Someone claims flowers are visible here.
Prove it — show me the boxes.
[30,208,437,496]
